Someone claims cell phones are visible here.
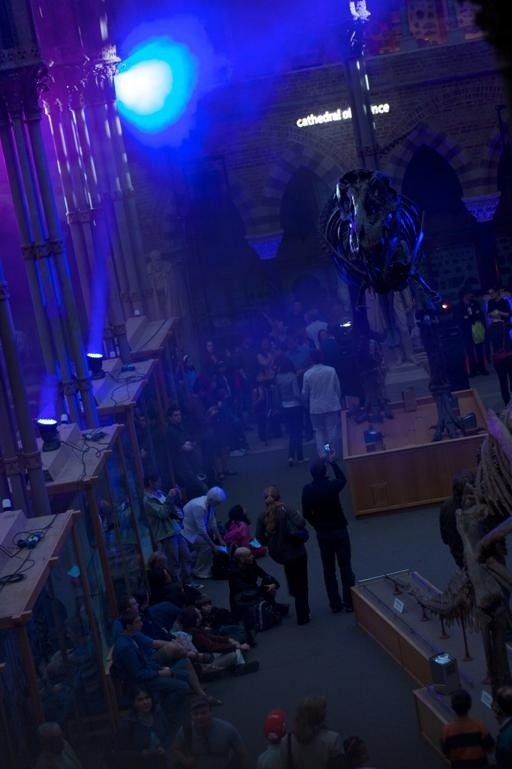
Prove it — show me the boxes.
[323,442,332,454]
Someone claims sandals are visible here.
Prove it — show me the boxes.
[204,695,224,706]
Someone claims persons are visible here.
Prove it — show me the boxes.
[37,282,512,769]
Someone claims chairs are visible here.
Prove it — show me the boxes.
[104,644,172,741]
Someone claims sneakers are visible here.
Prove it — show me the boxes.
[288,456,311,467]
[232,663,258,677]
[216,469,238,479]
[331,601,353,613]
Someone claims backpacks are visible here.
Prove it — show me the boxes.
[253,600,272,632]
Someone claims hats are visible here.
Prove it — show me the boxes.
[263,707,288,741]
[191,697,208,711]
[207,486,226,503]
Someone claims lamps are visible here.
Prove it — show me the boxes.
[34,418,62,453]
[85,352,108,381]
